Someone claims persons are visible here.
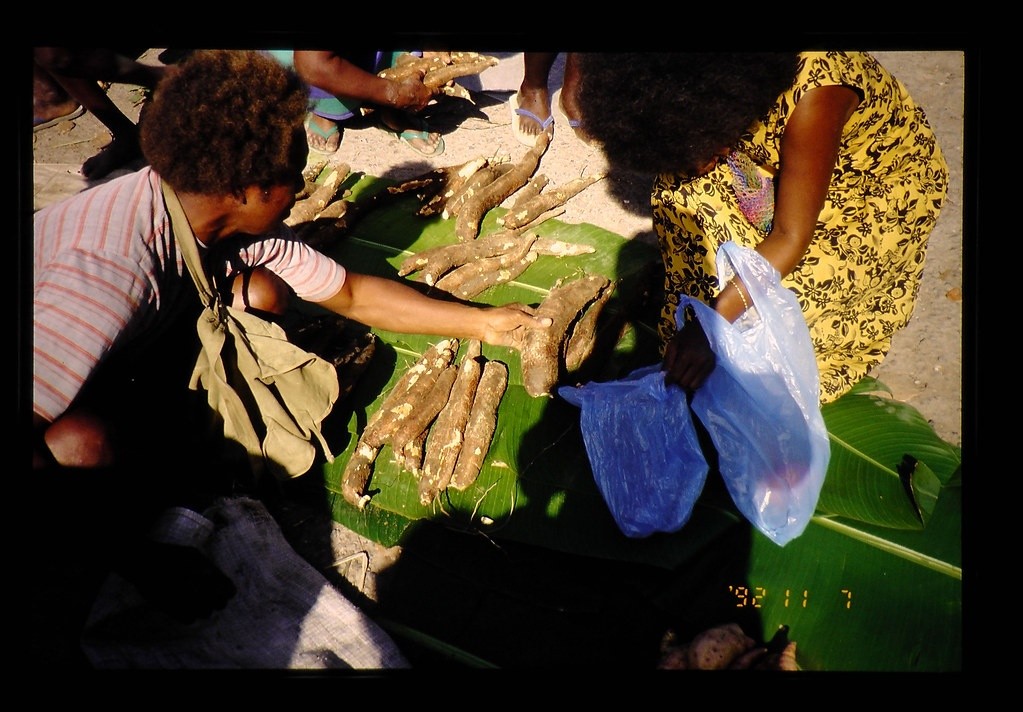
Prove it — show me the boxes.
[576,32,950,411]
[294,49,446,156]
[518,52,600,145]
[33,51,554,624]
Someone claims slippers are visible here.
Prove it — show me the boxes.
[307,118,338,155]
[509,94,554,148]
[33,105,84,132]
[559,104,605,150]
[380,118,444,157]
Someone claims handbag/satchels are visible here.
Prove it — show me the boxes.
[676,242,831,547]
[163,182,339,480]
[558,361,709,537]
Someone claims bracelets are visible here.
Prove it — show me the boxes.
[728,281,747,311]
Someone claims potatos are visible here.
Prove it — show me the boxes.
[282,53,612,497]
[653,623,798,671]
